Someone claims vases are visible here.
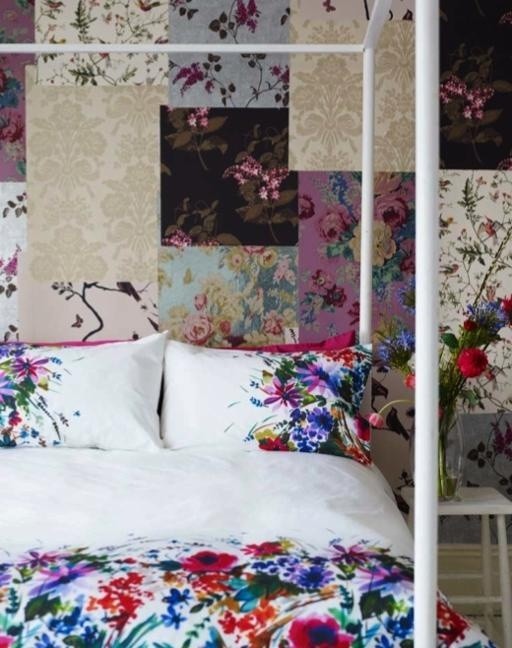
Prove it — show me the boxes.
[408,404,463,504]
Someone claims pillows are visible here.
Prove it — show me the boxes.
[161,339,374,466]
[0,329,170,450]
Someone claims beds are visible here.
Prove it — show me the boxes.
[0,432,465,648]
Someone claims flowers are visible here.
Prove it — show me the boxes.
[368,294,512,498]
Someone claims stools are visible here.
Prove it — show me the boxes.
[397,485,512,647]
